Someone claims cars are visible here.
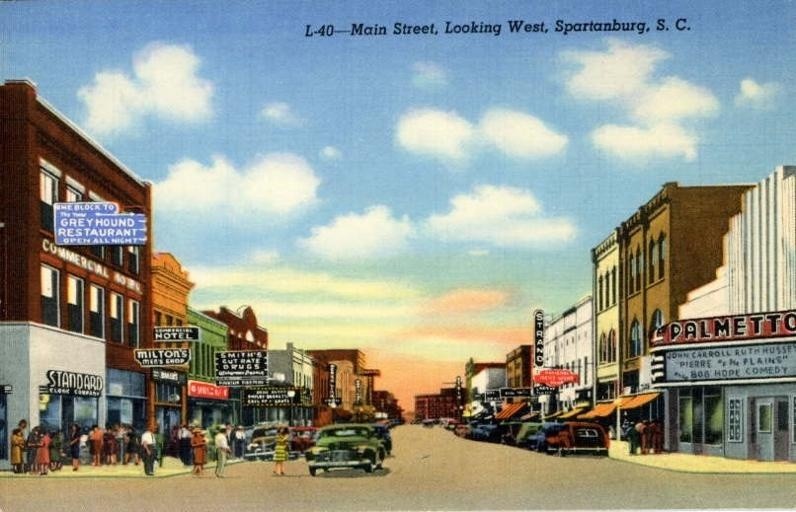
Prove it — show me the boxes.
[419,413,608,456]
[243,417,405,477]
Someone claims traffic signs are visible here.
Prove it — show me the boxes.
[51,200,146,248]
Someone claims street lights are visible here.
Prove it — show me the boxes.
[287,389,295,427]
[329,403,337,424]
[536,395,547,424]
[458,405,463,422]
